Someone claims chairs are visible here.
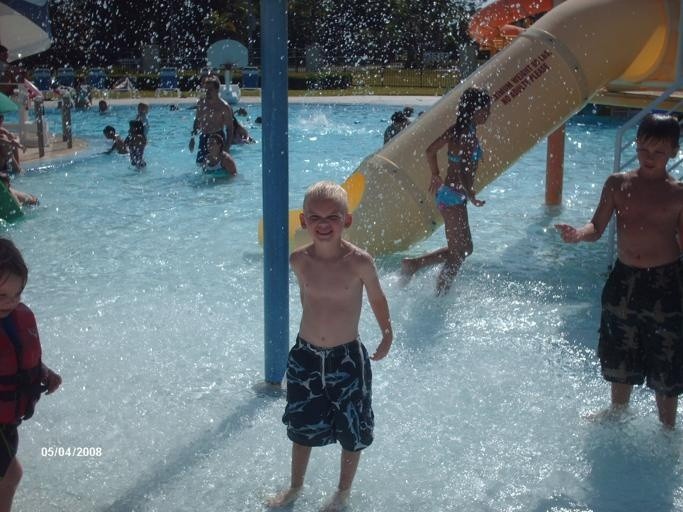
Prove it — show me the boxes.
[34,67,261,97]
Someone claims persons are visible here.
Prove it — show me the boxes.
[402,107,414,130]
[0,240,61,511]
[1,43,262,231]
[396,87,490,293]
[267,180,392,511]
[383,110,407,145]
[555,115,683,428]
